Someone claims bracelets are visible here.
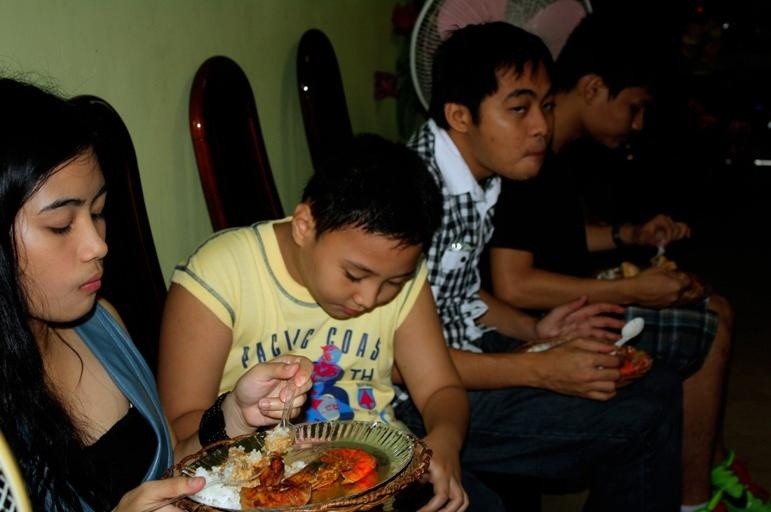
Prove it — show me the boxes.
[198,391,234,455]
[611,224,628,250]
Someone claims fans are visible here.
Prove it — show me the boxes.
[407,0,593,114]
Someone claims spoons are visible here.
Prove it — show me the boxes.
[598,316,646,371]
[651,240,667,262]
[268,374,297,454]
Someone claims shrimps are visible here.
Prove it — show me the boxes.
[239,444,380,509]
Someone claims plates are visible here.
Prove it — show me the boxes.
[177,421,417,512]
[596,264,698,298]
[525,337,650,374]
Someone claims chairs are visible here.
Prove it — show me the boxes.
[295,26,353,175]
[189,53,287,233]
[392,1,424,134]
[67,94,168,396]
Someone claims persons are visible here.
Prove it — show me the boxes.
[0,70,319,512]
[366,14,633,509]
[481,0,771,512]
[147,125,473,512]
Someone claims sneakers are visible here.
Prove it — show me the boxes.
[711,447,771,512]
